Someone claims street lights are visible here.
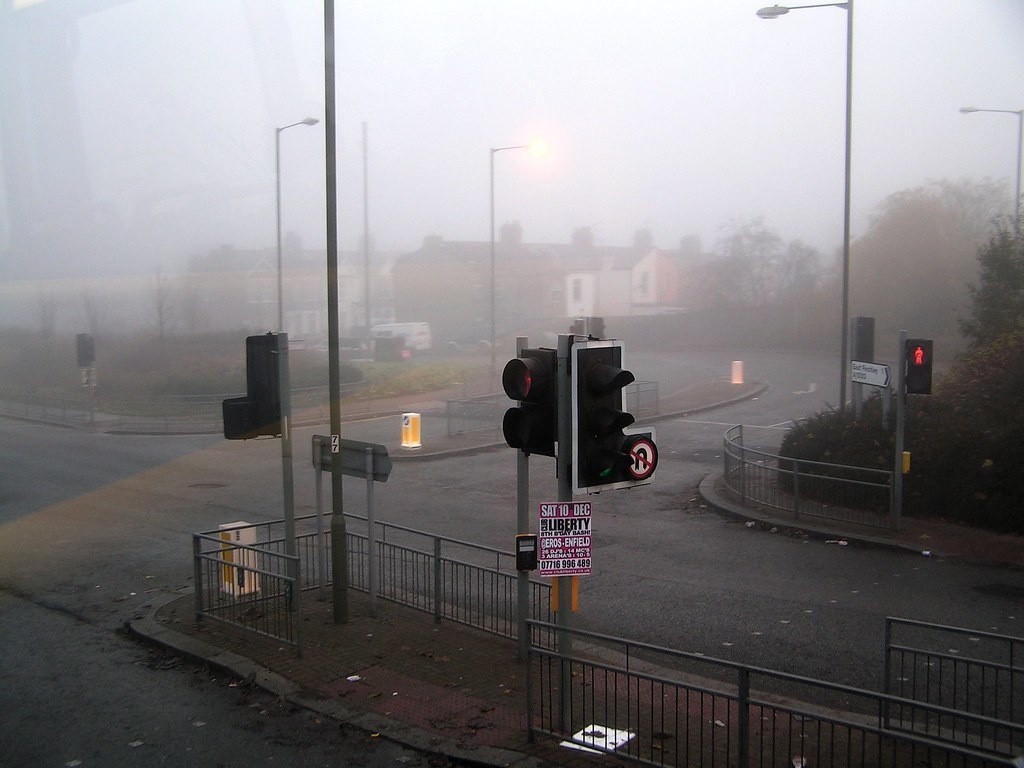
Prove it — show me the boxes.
[960,106,1024,240]
[486,143,528,402]
[750,0,857,415]
[275,117,324,614]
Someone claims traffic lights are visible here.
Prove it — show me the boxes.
[571,339,660,497]
[503,347,557,456]
[904,338,933,394]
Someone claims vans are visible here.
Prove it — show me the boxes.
[357,321,433,356]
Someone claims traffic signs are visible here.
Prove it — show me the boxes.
[851,360,891,387]
